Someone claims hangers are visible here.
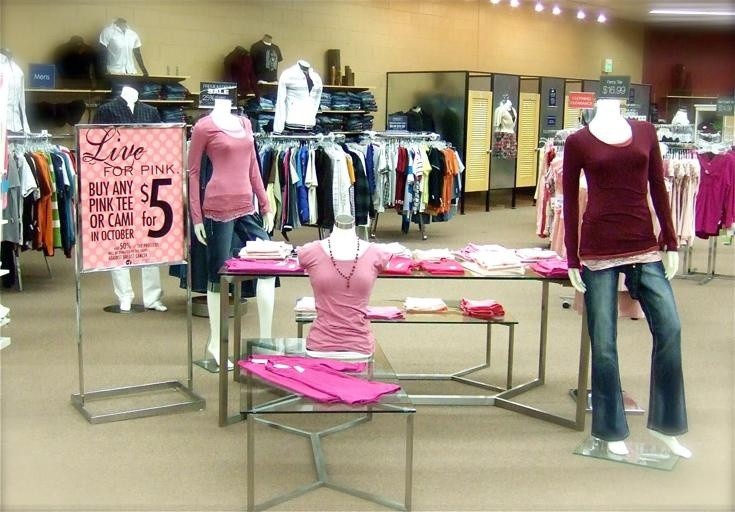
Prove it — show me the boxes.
[6,133,68,154]
[659,147,707,168]
[248,132,443,152]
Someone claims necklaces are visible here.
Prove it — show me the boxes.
[327,237,360,289]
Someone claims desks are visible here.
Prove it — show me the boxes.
[294,295,520,395]
[237,336,417,511]
[217,248,593,432]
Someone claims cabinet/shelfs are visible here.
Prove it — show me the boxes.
[110,70,198,107]
[250,81,371,138]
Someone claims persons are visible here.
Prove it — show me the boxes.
[671,108,688,126]
[98,18,147,76]
[297,215,378,360]
[94,87,169,312]
[224,46,258,100]
[495,100,518,160]
[250,35,283,83]
[272,60,323,134]
[55,35,98,89]
[562,99,693,458]
[187,99,276,367]
[1,48,29,135]
